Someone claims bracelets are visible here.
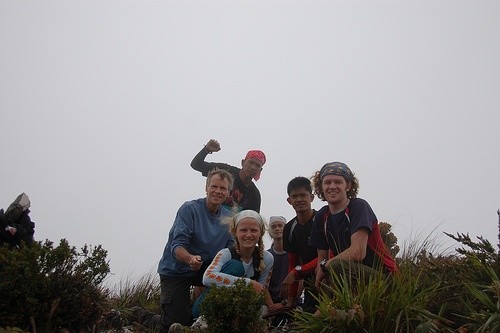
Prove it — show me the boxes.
[204,146,213,154]
[281,300,288,307]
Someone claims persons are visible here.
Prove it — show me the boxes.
[126,162,398,333]
[190,139,266,300]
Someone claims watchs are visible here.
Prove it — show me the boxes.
[295,265,303,277]
[319,259,330,276]
